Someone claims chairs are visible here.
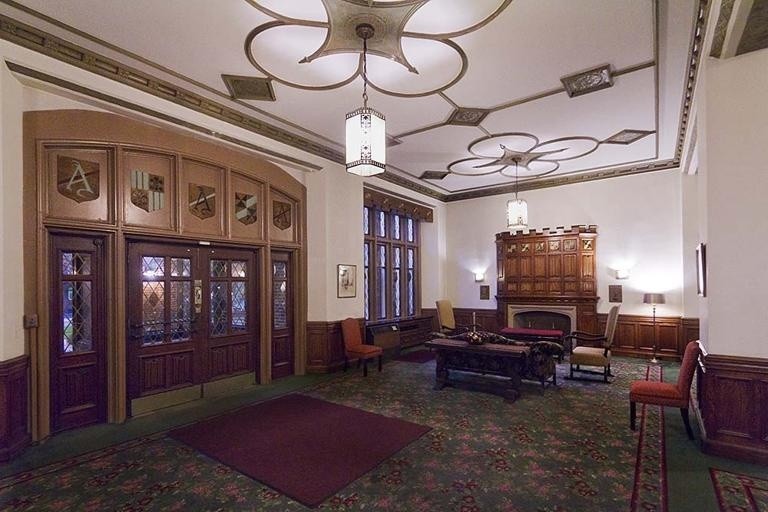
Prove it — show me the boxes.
[629,340,701,439]
[434,299,483,336]
[339,317,385,377]
[563,303,624,383]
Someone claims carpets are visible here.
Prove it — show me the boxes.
[1,329,672,511]
[704,463,768,512]
[166,390,434,510]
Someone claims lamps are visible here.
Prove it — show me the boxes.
[504,155,531,232]
[642,292,666,362]
[344,20,390,179]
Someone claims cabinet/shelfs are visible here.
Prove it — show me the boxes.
[424,338,534,404]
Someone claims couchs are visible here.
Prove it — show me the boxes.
[424,331,557,401]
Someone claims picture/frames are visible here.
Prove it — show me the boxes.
[694,242,707,299]
[336,263,358,300]
